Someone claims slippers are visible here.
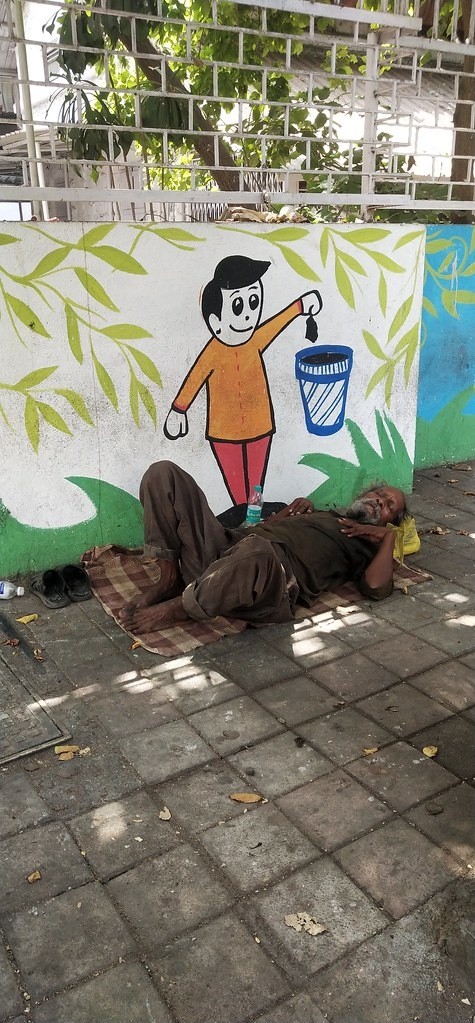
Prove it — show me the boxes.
[28,569,71,608]
[54,564,92,602]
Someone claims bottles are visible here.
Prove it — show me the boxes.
[246,485,263,527]
[0,581,24,599]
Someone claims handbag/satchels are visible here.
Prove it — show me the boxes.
[385,515,422,560]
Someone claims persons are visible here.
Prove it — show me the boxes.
[119,462,407,635]
